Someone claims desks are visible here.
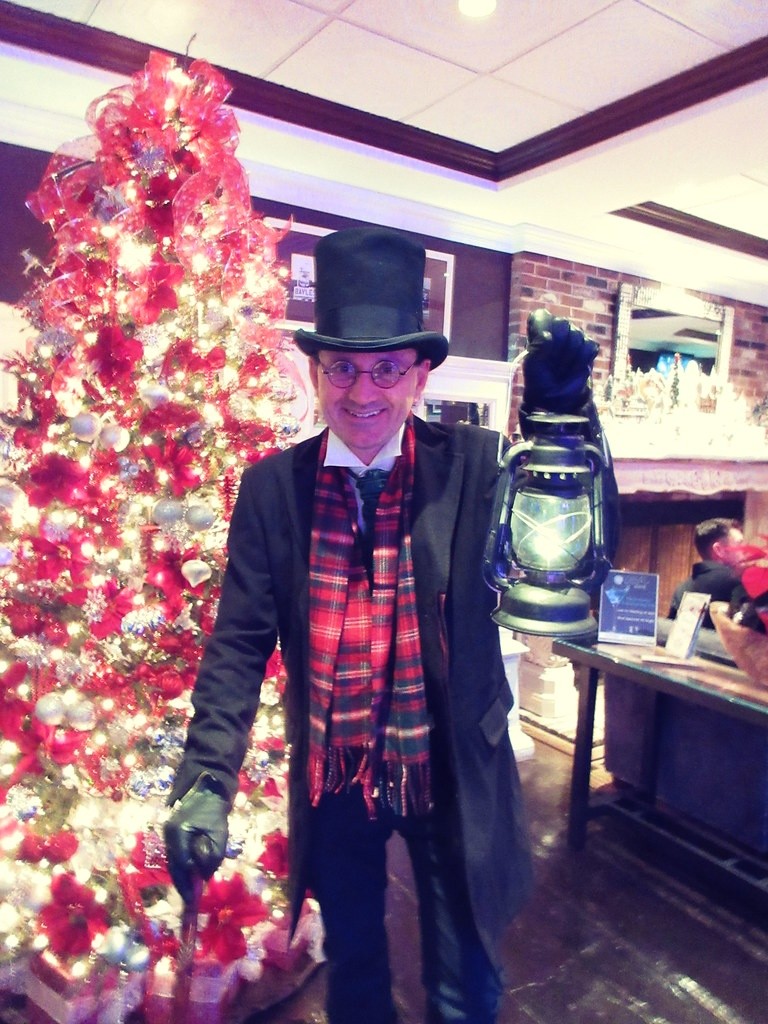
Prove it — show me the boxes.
[552,634,768,898]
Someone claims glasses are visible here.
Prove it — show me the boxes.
[318,358,423,389]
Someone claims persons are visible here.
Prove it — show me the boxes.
[668,519,750,629]
[164,224,620,1024]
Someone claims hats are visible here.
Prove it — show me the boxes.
[293,227,449,373]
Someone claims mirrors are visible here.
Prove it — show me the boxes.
[613,284,736,415]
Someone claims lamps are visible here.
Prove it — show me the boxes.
[486,349,622,638]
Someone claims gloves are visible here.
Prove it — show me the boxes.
[163,782,228,905]
[520,310,600,414]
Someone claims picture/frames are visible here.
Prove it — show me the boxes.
[263,217,465,364]
